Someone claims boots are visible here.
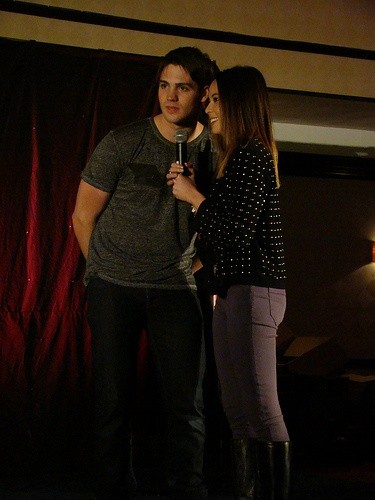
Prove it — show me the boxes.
[230,437,260,500]
[256,440,293,500]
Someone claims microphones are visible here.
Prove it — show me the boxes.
[174,128,188,176]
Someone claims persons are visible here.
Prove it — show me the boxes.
[70,45,223,500]
[163,66,296,500]
